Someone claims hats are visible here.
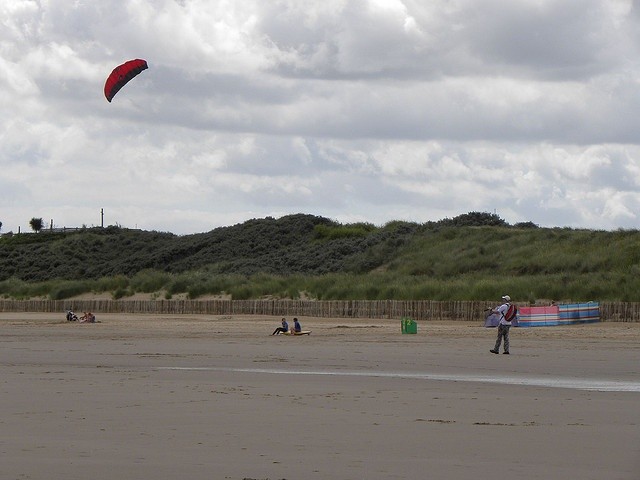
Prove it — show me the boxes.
[502,295,510,300]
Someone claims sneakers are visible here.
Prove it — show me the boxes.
[490,350,499,354]
[503,351,509,353]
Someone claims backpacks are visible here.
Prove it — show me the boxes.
[500,304,517,321]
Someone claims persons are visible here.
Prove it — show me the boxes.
[66,309,79,323]
[80,311,95,324]
[272,318,288,335]
[290,318,301,335]
[487,295,512,355]
